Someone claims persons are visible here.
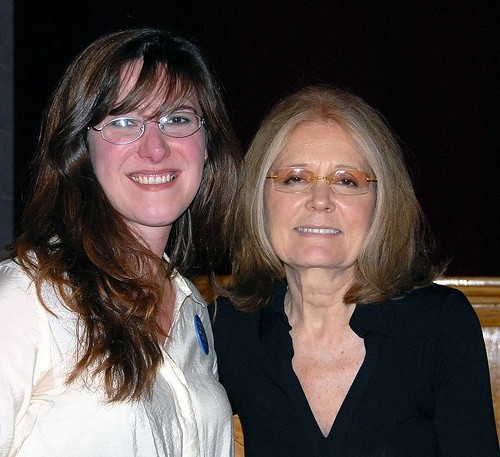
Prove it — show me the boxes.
[210,84,500,457]
[0,29,247,457]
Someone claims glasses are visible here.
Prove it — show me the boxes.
[89,111,208,144]
[264,168,379,194]
